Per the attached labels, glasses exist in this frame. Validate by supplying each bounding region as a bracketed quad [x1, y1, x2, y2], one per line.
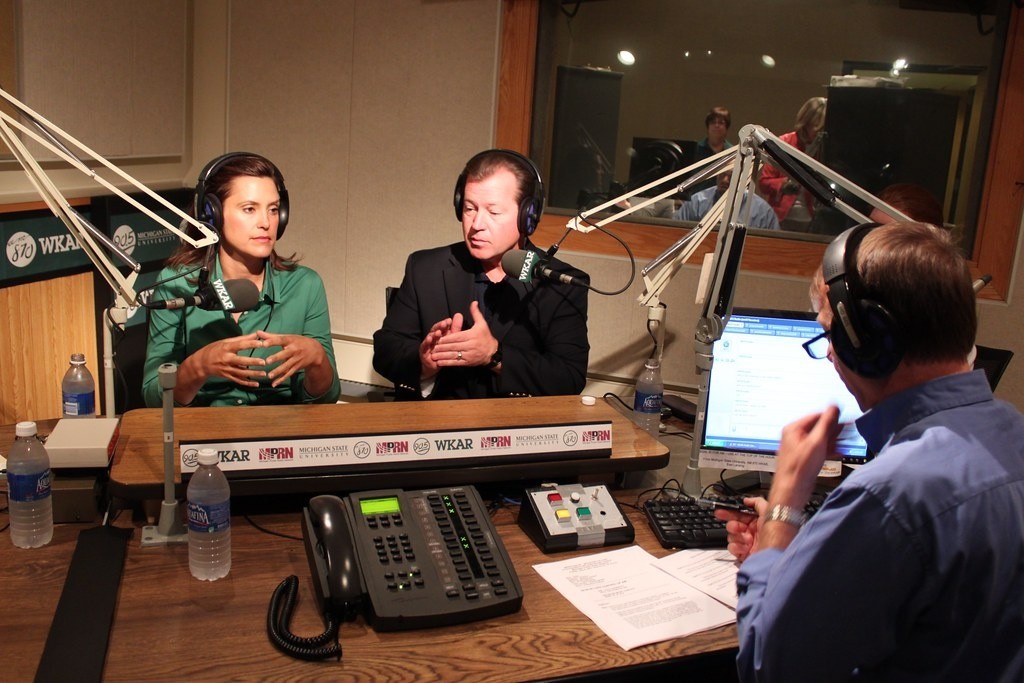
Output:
[802, 322, 834, 363]
[710, 120, 727, 125]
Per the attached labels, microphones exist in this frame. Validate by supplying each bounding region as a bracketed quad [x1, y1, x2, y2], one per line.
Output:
[149, 279, 261, 313]
[501, 250, 585, 287]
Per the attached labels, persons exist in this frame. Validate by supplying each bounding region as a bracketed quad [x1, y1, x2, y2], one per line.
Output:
[692, 105, 733, 193]
[672, 149, 781, 231]
[615, 194, 676, 220]
[372, 150, 591, 395]
[141, 150, 341, 409]
[758, 95, 828, 233]
[715, 222, 1024, 683]
[871, 181, 946, 229]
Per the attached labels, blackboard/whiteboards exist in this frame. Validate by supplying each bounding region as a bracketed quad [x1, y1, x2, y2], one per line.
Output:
[0, 0, 194, 206]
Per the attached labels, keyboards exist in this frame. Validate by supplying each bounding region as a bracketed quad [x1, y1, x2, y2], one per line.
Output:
[643, 494, 825, 550]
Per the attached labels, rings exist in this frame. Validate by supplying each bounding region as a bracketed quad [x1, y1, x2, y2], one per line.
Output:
[458, 351, 462, 360]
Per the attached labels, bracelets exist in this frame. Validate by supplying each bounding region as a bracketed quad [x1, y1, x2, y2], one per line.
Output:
[767, 503, 809, 528]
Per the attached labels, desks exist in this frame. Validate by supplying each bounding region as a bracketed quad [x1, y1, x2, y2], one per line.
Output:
[0, 396, 851, 683]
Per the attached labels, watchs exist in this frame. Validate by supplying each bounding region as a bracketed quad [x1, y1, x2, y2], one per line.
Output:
[487, 349, 501, 369]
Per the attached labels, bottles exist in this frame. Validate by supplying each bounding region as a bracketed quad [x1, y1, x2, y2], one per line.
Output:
[186, 448, 231, 581]
[631, 359, 663, 440]
[62, 354, 96, 419]
[7, 421, 53, 549]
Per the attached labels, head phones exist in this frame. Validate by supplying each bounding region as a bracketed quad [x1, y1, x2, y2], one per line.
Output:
[454, 149, 544, 237]
[821, 222, 908, 379]
[194, 151, 290, 241]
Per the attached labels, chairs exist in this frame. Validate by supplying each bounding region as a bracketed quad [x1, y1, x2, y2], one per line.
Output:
[114, 273, 153, 409]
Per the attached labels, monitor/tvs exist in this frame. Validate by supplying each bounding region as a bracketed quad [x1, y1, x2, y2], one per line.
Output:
[697, 306, 874, 498]
[629, 137, 698, 201]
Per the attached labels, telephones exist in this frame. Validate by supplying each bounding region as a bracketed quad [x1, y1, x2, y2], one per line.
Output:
[301, 484, 524, 632]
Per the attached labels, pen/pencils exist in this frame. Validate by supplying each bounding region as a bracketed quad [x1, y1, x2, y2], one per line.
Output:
[688, 497, 759, 516]
